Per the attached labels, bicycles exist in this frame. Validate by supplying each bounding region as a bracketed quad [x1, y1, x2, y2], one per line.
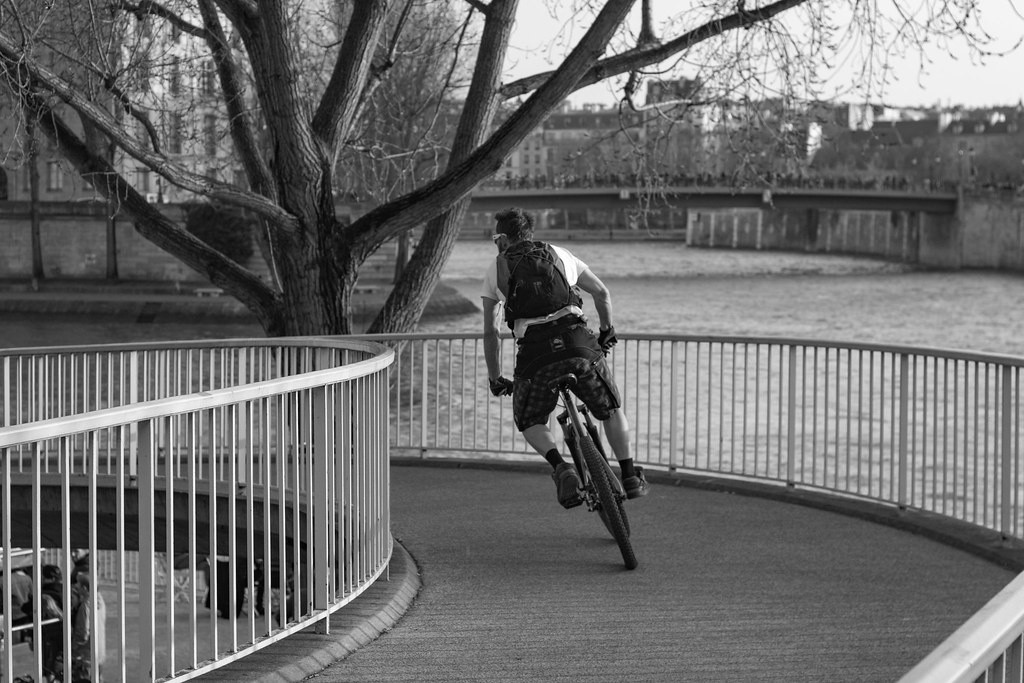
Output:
[500, 338, 639, 569]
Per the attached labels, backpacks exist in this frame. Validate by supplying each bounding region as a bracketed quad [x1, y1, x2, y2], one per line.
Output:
[503, 240, 584, 338]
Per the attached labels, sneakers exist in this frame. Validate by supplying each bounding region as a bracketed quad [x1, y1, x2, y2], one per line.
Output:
[622, 466, 648, 499]
[551, 462, 584, 510]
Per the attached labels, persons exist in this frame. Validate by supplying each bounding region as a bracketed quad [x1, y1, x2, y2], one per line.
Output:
[480, 206, 650, 508]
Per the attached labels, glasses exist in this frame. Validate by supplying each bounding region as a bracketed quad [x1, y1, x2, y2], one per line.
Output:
[492, 233, 508, 244]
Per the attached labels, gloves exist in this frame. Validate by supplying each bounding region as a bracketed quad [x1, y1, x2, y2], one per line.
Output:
[597, 326, 618, 354]
[489, 376, 513, 396]
